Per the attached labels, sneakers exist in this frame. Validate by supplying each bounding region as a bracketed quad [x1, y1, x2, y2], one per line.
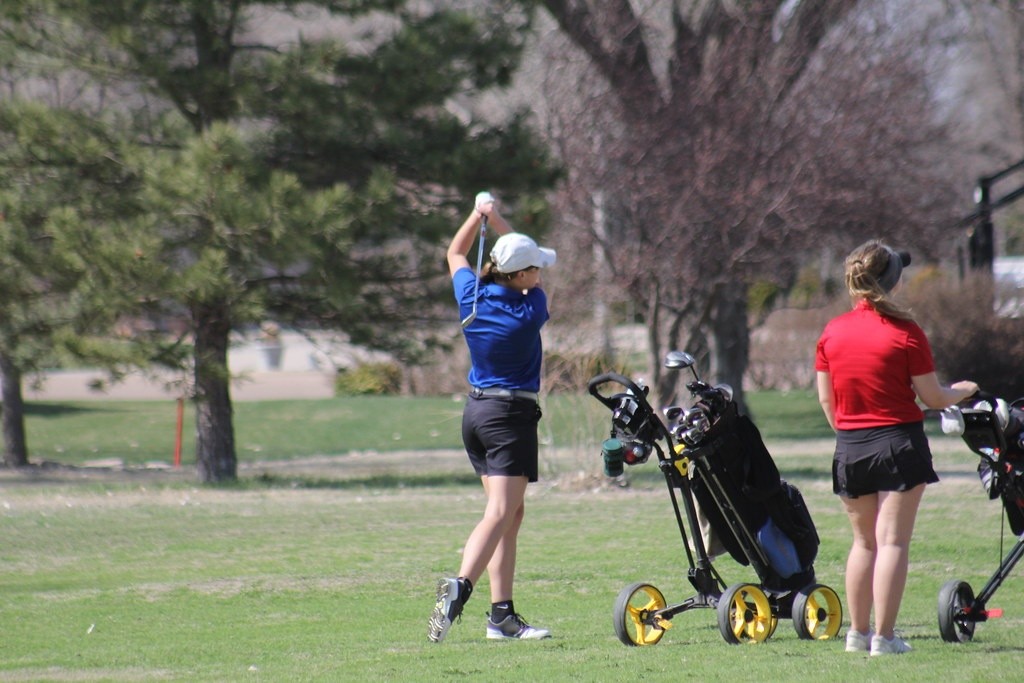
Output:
[845, 630, 876, 652]
[427, 577, 465, 644]
[485, 612, 552, 641]
[869, 635, 915, 656]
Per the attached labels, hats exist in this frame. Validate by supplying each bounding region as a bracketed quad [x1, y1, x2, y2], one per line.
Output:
[489, 233, 557, 273]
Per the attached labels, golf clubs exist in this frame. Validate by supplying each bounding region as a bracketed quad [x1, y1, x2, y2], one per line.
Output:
[460, 214, 488, 328]
[660, 351, 726, 446]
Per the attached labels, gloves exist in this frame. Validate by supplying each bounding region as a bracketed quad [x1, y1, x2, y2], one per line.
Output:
[474, 191, 495, 210]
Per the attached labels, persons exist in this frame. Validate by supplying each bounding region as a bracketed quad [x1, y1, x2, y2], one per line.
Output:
[425, 190, 558, 644]
[813, 239, 982, 658]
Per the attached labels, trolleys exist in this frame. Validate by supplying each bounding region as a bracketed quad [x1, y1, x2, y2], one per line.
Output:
[926, 384, 1024, 643]
[585, 374, 843, 649]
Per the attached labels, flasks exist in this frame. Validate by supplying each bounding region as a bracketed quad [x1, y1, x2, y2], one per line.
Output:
[602, 438, 624, 479]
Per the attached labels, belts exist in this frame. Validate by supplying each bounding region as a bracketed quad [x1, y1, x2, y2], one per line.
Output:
[470, 385, 539, 402]
